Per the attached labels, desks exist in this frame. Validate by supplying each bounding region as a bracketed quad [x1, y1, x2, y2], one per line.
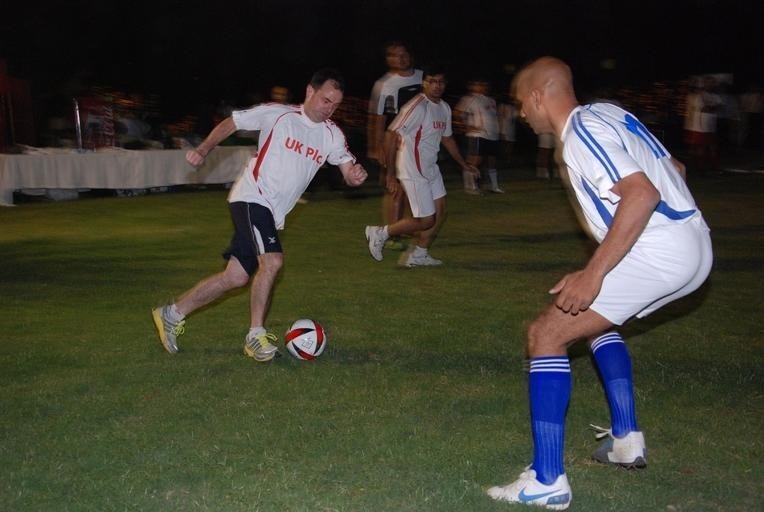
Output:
[0, 142, 255, 206]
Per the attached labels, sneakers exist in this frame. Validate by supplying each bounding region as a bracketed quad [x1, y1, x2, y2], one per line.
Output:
[151, 304, 185, 355]
[589, 424, 647, 471]
[365, 225, 385, 261]
[486, 463, 572, 510]
[406, 253, 443, 267]
[242, 334, 282, 362]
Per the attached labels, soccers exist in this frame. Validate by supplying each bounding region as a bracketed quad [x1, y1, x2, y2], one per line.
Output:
[283, 318, 327, 361]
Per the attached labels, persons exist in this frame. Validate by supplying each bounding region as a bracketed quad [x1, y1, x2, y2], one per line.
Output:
[364, 67, 482, 268]
[149, 67, 369, 363]
[364, 41, 426, 251]
[484, 57, 714, 510]
[452, 77, 504, 198]
[683, 76, 764, 177]
[268, 81, 289, 105]
[535, 133, 560, 179]
